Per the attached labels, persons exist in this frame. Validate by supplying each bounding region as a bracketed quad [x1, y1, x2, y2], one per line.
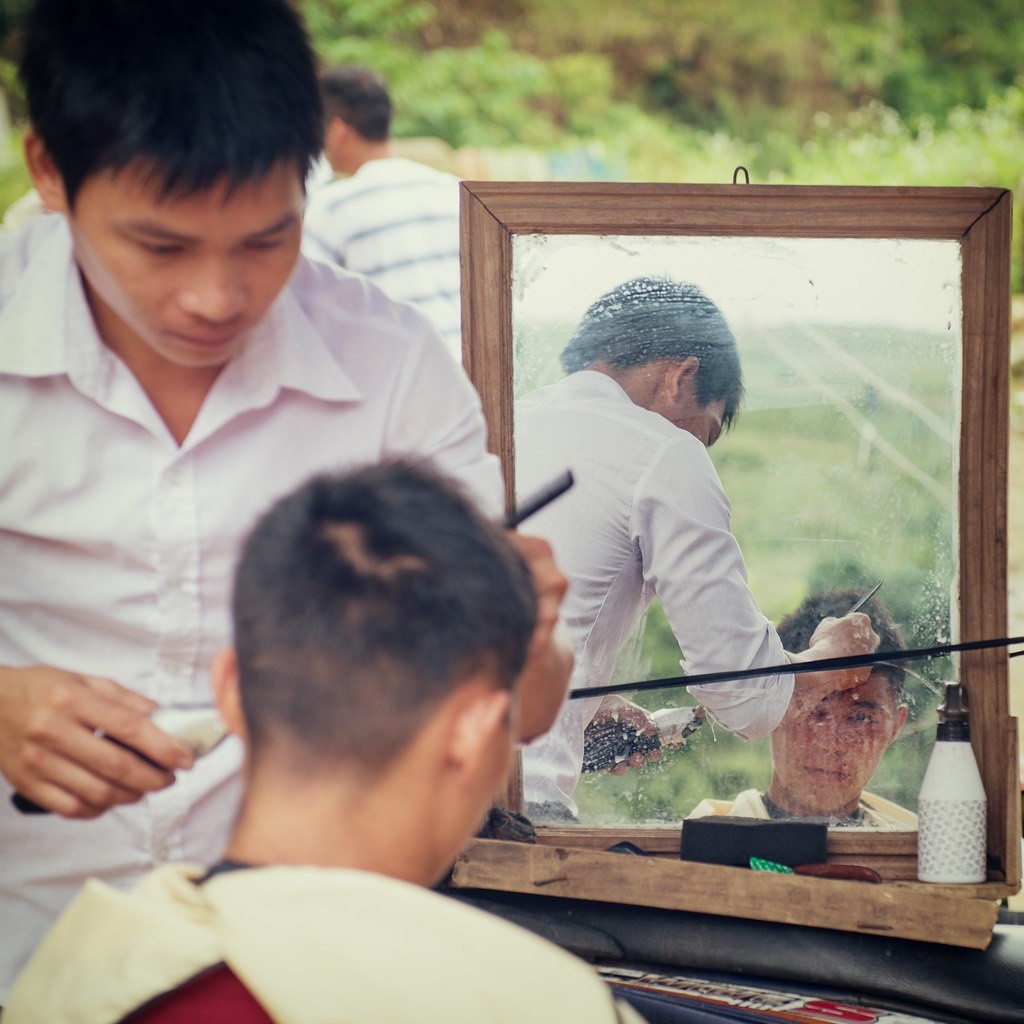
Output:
[684, 588, 912, 831]
[0, 0, 578, 1012]
[298, 64, 459, 364]
[1, 446, 636, 1024]
[514, 271, 883, 820]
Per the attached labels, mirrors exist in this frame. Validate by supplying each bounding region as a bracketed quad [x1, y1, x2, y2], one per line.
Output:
[457, 181, 1013, 881]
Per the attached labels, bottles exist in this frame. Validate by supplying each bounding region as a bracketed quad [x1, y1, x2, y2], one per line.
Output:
[917, 686, 989, 885]
